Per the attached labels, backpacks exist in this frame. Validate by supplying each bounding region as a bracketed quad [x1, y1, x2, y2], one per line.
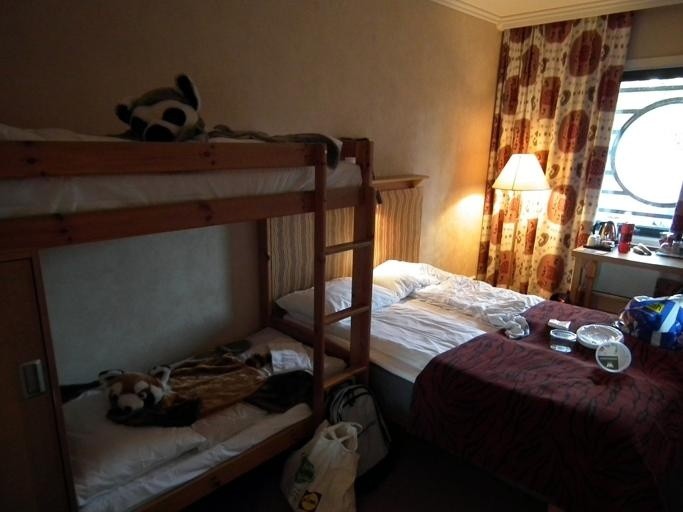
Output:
[324, 380, 392, 477]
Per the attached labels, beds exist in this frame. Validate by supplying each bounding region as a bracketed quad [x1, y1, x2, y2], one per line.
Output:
[0, 135, 378, 512]
[258, 175, 682, 511]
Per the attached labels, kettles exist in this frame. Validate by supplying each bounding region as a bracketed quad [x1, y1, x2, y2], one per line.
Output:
[592, 220, 619, 246]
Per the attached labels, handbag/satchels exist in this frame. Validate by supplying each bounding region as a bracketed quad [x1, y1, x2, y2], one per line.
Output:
[276, 420, 364, 512]
[611, 294, 683, 349]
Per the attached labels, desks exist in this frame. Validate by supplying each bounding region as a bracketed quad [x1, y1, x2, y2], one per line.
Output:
[568, 239, 682, 316]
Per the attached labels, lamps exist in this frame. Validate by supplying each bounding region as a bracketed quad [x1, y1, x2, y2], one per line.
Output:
[491, 153, 552, 290]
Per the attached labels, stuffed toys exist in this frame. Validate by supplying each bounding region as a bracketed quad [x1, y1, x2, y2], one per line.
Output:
[107, 72, 206, 143]
[97, 364, 204, 427]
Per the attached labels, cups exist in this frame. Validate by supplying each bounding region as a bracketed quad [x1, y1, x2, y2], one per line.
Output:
[586, 234, 615, 246]
[616, 222, 635, 253]
[548, 329, 577, 353]
[658, 243, 669, 253]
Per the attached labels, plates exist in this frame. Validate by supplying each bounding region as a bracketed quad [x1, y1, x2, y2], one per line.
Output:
[575, 324, 625, 350]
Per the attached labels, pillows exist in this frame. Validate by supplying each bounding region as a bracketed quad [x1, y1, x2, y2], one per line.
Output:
[274, 274, 400, 327]
[61, 387, 206, 506]
[373, 259, 452, 301]
